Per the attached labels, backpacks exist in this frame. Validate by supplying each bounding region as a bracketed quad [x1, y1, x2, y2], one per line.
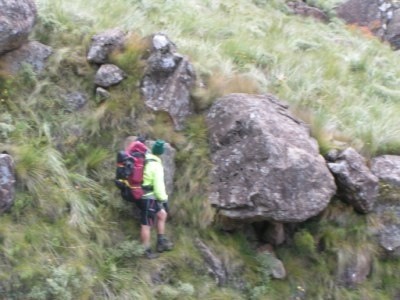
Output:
[117, 141, 147, 200]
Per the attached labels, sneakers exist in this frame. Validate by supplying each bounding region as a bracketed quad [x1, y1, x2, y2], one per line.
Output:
[144, 251, 159, 259]
[157, 241, 174, 252]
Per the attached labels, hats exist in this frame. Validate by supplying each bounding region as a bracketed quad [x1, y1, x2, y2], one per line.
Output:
[152, 140, 165, 154]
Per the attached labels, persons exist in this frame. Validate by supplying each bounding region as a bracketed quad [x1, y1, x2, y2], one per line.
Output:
[142, 141, 175, 260]
